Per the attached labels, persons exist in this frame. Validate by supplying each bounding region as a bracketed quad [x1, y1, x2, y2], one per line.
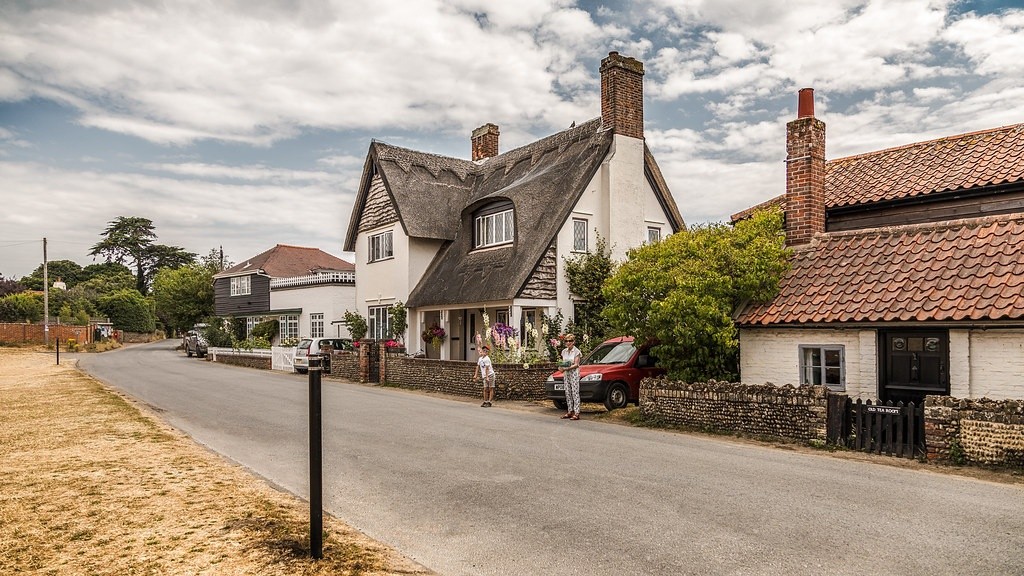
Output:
[558, 333, 583, 420]
[472, 345, 495, 407]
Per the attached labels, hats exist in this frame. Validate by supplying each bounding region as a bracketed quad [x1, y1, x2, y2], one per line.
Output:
[481, 344, 491, 350]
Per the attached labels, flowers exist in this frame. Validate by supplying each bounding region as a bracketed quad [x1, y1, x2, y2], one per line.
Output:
[385, 338, 404, 347]
[489, 323, 516, 348]
[421, 323, 447, 353]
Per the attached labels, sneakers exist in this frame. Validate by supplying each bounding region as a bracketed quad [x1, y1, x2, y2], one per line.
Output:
[481, 401, 492, 407]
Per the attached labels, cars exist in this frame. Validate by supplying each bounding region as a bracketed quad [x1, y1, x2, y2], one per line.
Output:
[99, 327, 110, 338]
[543, 334, 668, 412]
[293, 337, 352, 374]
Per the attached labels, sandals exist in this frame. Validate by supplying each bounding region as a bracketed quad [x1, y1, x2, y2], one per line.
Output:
[570, 413, 580, 420]
[560, 412, 572, 419]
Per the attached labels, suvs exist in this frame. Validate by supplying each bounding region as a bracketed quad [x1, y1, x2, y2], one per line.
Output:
[185, 330, 211, 358]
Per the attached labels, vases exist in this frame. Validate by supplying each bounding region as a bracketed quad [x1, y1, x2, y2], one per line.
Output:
[387, 347, 405, 352]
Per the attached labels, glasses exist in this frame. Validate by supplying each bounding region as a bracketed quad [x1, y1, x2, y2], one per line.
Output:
[565, 340, 574, 342]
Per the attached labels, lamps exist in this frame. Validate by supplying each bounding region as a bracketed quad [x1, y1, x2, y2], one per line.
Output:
[458, 315, 462, 326]
